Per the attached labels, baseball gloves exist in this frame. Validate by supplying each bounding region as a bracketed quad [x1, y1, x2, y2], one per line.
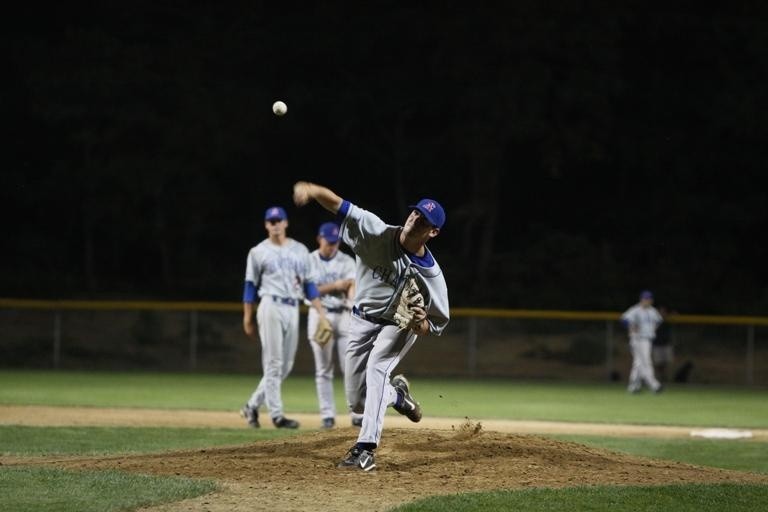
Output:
[313, 318, 334, 347]
[392, 279, 426, 332]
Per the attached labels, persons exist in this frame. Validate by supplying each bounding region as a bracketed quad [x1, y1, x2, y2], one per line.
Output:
[304, 217, 363, 432]
[617, 288, 668, 396]
[290, 177, 451, 473]
[651, 304, 681, 384]
[238, 203, 334, 430]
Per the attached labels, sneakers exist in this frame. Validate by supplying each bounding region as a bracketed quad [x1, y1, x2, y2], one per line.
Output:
[337, 443, 378, 472]
[350, 416, 362, 427]
[239, 404, 262, 429]
[321, 417, 335, 428]
[390, 373, 422, 423]
[271, 416, 300, 430]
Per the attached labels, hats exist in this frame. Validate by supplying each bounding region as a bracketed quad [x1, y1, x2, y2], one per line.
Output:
[318, 222, 341, 243]
[408, 199, 446, 229]
[640, 291, 653, 299]
[264, 206, 287, 221]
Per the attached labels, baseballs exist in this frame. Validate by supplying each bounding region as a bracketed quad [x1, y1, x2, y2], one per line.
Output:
[273, 101, 288, 117]
